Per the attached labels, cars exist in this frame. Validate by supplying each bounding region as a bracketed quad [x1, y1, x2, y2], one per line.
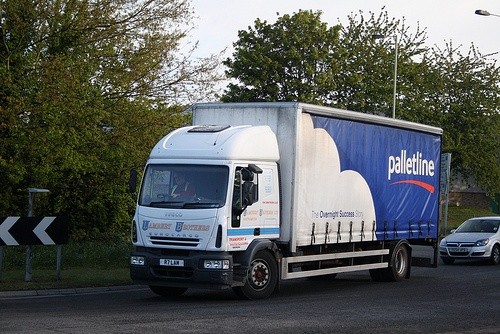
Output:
[440, 216, 500, 265]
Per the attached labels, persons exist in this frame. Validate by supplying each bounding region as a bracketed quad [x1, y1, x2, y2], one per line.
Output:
[168, 171, 195, 199]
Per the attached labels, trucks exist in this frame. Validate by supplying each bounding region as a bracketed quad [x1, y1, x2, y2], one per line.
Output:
[132, 103, 442, 302]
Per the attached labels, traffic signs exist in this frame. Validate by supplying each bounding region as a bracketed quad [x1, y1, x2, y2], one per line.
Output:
[0, 216, 70, 245]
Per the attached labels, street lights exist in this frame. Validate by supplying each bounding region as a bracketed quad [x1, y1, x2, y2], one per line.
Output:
[372, 33, 400, 119]
